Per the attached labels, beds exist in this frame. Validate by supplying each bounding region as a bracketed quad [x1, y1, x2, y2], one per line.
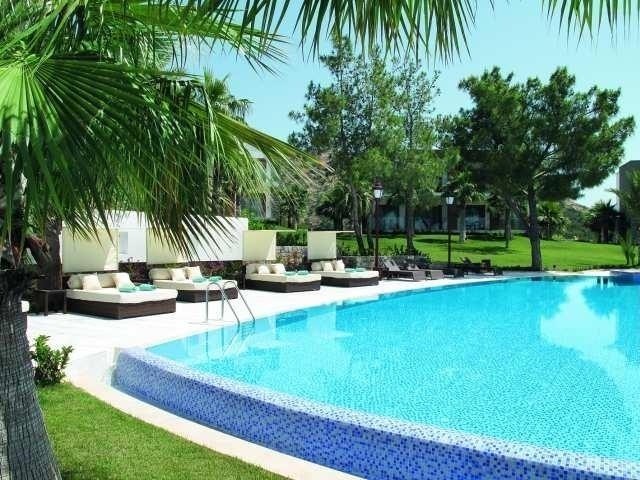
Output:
[148, 267, 238, 303]
[62, 270, 179, 319]
[309, 261, 379, 287]
[244, 263, 321, 293]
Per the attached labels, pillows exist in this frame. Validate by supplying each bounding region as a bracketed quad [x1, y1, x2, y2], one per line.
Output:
[320, 260, 344, 272]
[255, 263, 286, 274]
[167, 266, 202, 282]
[77, 271, 137, 292]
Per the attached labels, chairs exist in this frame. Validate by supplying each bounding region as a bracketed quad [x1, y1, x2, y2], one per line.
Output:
[382, 255, 445, 282]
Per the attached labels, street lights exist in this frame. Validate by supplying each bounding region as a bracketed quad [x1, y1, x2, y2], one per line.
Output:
[370, 178, 385, 282]
[442, 190, 457, 274]
